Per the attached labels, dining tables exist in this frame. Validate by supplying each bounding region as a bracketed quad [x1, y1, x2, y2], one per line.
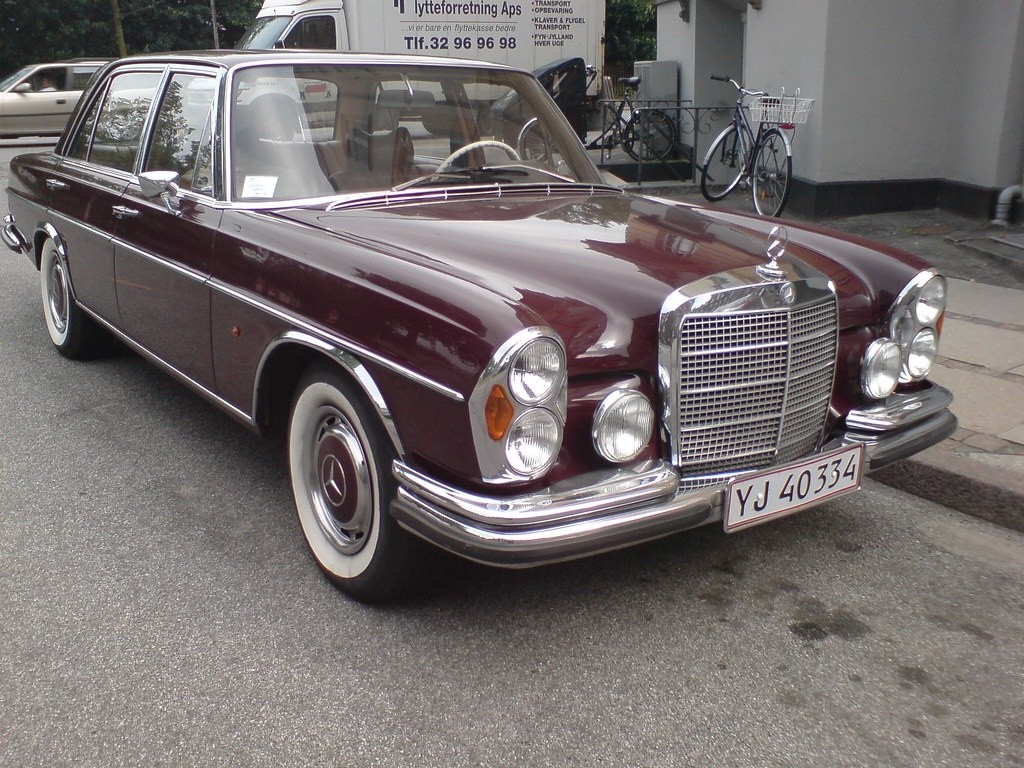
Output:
[349, 127, 422, 191]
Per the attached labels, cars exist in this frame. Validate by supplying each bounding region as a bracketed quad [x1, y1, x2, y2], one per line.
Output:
[2, 46, 962, 603]
[0, 59, 157, 141]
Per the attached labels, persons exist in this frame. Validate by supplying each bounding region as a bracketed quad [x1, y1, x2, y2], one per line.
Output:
[38, 73, 58, 92]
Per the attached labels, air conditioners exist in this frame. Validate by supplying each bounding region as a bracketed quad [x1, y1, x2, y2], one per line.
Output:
[633, 60, 678, 158]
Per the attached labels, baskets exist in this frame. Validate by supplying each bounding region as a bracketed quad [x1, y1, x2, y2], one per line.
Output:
[747, 94, 813, 124]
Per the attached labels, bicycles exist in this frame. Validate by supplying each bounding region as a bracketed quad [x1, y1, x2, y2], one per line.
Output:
[518, 62, 677, 168]
[700, 73, 813, 217]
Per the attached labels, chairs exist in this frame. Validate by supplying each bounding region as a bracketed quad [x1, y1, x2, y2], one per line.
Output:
[237, 106, 299, 197]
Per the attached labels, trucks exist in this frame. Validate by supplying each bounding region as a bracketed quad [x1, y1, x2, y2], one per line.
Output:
[189, 0, 609, 161]
